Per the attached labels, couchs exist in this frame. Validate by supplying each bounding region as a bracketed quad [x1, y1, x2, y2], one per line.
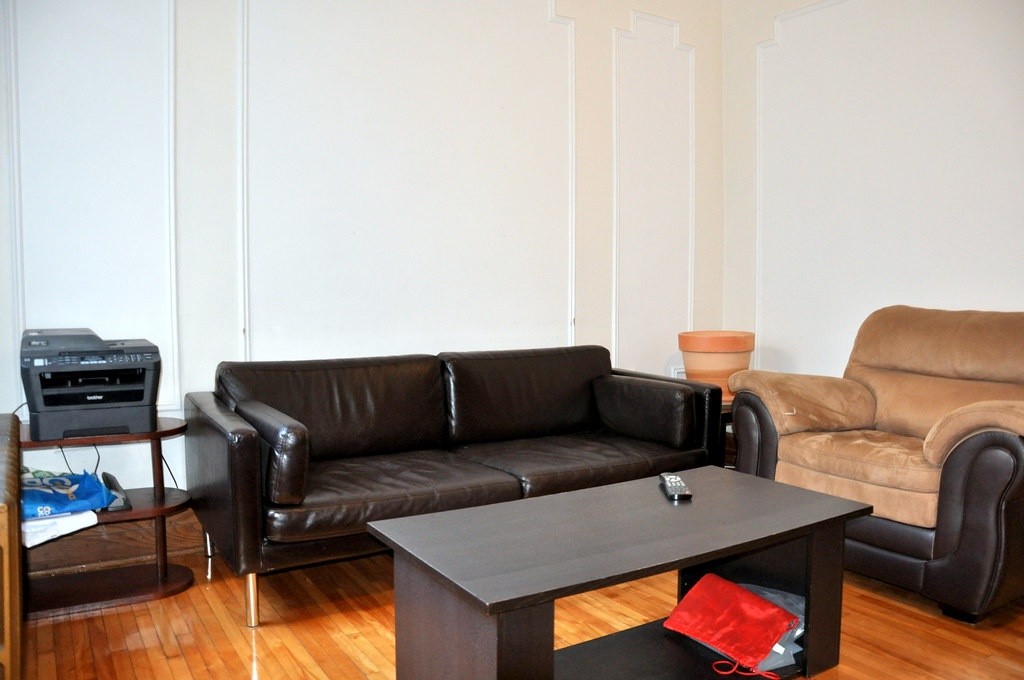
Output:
[728, 305, 1024, 626]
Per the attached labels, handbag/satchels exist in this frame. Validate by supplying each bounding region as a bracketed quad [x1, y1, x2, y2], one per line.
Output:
[663, 572, 806, 680]
[19, 466, 118, 548]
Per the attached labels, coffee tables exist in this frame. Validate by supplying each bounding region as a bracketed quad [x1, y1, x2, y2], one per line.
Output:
[361, 465, 875, 680]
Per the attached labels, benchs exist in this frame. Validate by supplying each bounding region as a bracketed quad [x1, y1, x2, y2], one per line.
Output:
[183, 344, 726, 628]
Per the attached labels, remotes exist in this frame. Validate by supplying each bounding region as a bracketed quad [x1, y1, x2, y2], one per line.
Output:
[659, 472, 692, 501]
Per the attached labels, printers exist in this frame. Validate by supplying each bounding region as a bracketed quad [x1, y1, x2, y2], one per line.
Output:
[21, 328, 162, 442]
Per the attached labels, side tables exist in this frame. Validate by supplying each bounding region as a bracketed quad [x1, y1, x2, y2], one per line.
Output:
[18, 416, 195, 622]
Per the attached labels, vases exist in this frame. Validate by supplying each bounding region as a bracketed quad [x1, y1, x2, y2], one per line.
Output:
[678, 331, 756, 404]
